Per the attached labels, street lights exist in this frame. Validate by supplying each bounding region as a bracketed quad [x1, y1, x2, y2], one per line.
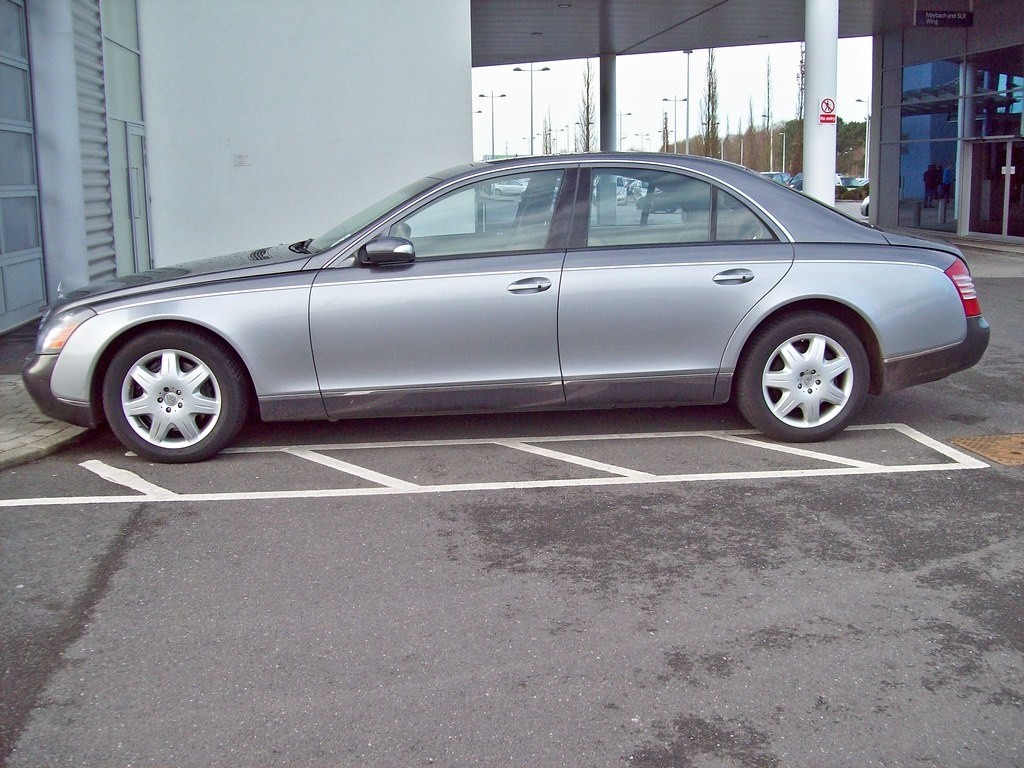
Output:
[684, 49, 694, 153]
[635, 133, 648, 150]
[703, 122, 721, 156]
[574, 122, 580, 153]
[549, 128, 564, 153]
[662, 99, 688, 153]
[566, 125, 570, 152]
[763, 115, 773, 172]
[616, 113, 632, 151]
[479, 94, 506, 158]
[514, 66, 551, 155]
[546, 133, 551, 154]
[646, 138, 649, 149]
[779, 133, 786, 172]
[658, 130, 673, 153]
[523, 137, 536, 156]
[855, 98, 870, 178]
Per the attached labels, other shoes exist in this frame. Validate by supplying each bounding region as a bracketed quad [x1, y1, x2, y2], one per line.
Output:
[923, 205, 927, 208]
[929, 205, 934, 208]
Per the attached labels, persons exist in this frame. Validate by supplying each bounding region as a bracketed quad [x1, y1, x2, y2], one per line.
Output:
[924, 164, 943, 208]
[943, 161, 953, 204]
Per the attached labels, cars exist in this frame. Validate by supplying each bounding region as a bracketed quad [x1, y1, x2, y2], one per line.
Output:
[624, 178, 662, 197]
[636, 188, 685, 213]
[524, 178, 561, 199]
[835, 173, 869, 191]
[23, 147, 992, 462]
[861, 196, 870, 216]
[491, 179, 529, 194]
[593, 176, 628, 204]
[757, 170, 802, 191]
